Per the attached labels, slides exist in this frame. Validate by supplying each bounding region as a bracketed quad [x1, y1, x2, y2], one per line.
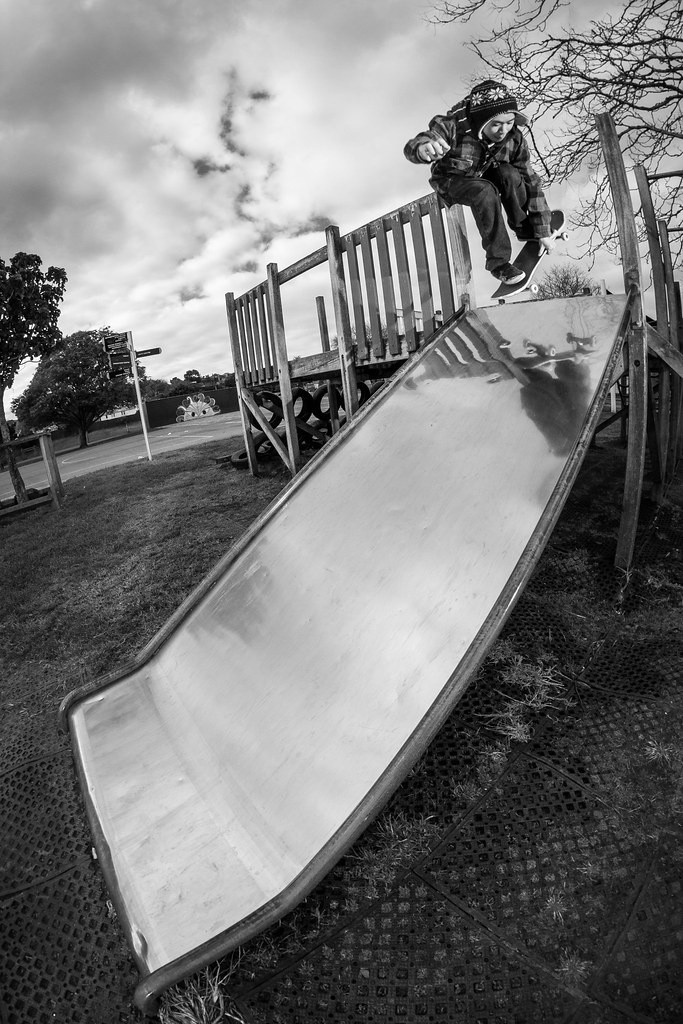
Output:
[60, 295, 634, 1012]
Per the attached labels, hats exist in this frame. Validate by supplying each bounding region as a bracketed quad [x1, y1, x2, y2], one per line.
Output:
[469, 80, 550, 189]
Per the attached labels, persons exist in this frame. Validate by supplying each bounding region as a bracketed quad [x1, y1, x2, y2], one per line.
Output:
[405, 79, 551, 285]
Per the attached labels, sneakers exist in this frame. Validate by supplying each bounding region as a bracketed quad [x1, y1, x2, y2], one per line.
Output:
[491, 262, 526, 285]
[517, 227, 557, 241]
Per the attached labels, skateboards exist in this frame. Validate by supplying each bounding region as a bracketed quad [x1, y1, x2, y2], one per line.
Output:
[492, 208, 568, 300]
